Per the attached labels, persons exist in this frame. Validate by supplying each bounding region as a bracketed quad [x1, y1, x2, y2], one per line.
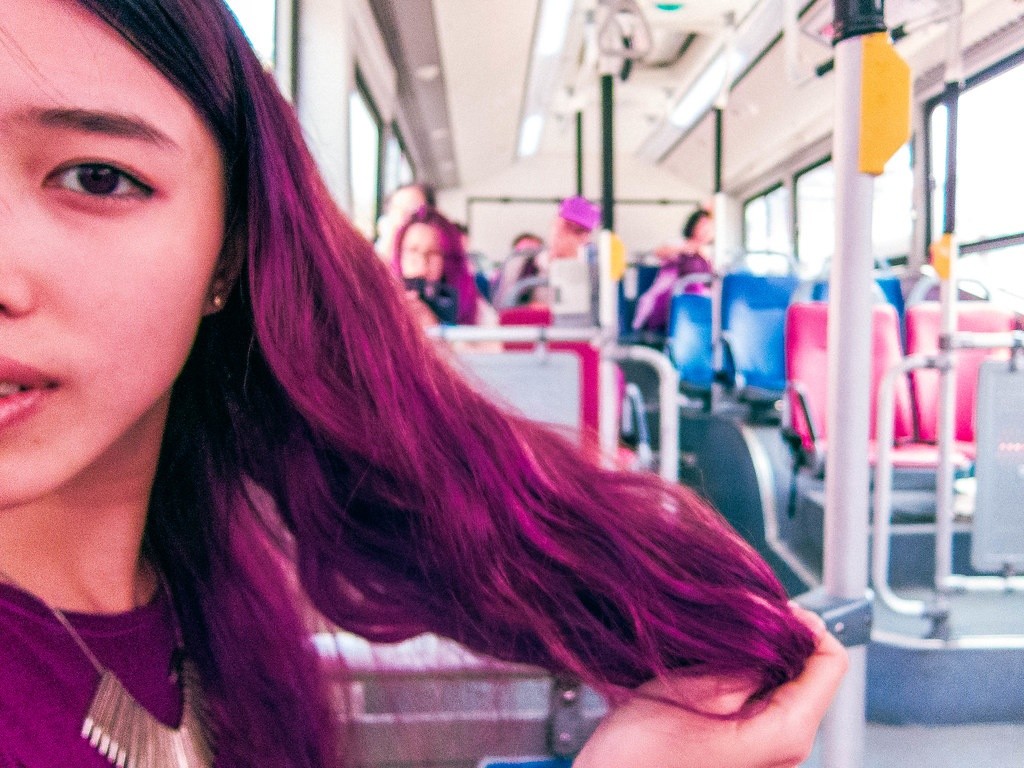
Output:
[0, 0, 847, 768]
[371, 184, 436, 264]
[682, 210, 713, 261]
[396, 208, 498, 327]
[493, 196, 614, 306]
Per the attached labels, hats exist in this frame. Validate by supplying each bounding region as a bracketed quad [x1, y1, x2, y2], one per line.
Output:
[559, 196, 601, 230]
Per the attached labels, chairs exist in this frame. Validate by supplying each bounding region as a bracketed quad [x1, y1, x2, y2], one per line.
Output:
[312, 248, 1023, 768]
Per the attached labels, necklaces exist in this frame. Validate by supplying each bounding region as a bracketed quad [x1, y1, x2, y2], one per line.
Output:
[0, 570, 219, 768]
